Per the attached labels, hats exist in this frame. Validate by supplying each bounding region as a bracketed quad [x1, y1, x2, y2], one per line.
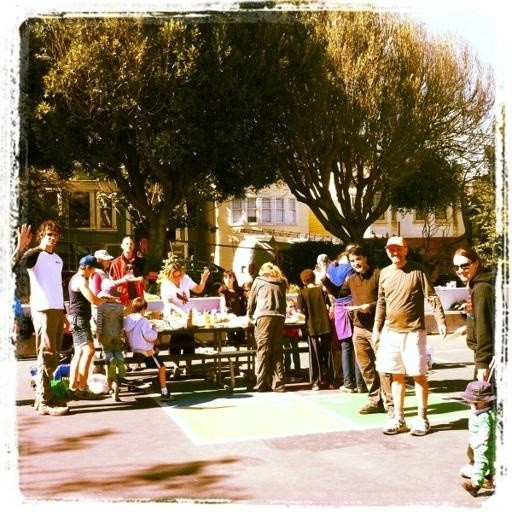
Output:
[79, 255, 103, 269]
[459, 380, 496, 403]
[385, 235, 407, 248]
[94, 249, 115, 261]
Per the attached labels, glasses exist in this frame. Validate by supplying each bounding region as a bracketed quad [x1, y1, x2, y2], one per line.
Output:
[450, 260, 475, 270]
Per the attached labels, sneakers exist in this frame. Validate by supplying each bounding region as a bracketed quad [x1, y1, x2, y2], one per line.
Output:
[339, 385, 355, 393]
[312, 385, 320, 391]
[482, 479, 493, 488]
[70, 386, 104, 401]
[383, 420, 409, 435]
[329, 384, 336, 389]
[410, 418, 431, 436]
[460, 463, 474, 479]
[459, 477, 480, 497]
[388, 405, 394, 418]
[34, 398, 70, 416]
[173, 367, 180, 379]
[160, 393, 176, 401]
[357, 403, 386, 414]
[184, 368, 195, 378]
[356, 387, 368, 393]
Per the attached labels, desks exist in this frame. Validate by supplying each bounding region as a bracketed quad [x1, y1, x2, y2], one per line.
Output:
[70, 310, 312, 371]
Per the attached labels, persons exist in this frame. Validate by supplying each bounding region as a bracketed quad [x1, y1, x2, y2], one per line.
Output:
[371, 237, 448, 436]
[458, 375, 495, 497]
[452, 247, 495, 479]
[11, 220, 69, 415]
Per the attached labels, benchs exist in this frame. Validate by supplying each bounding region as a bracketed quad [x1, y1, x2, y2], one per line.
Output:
[92, 344, 309, 402]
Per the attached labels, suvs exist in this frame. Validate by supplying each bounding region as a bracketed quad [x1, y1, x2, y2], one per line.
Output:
[129, 256, 238, 288]
